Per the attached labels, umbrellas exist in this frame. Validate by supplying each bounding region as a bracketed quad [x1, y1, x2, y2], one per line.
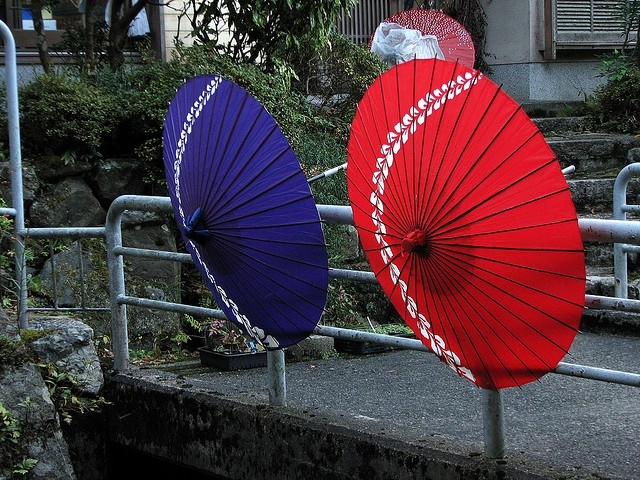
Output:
[347, 54, 590, 393]
[367, 9, 476, 73]
[161, 73, 330, 350]
